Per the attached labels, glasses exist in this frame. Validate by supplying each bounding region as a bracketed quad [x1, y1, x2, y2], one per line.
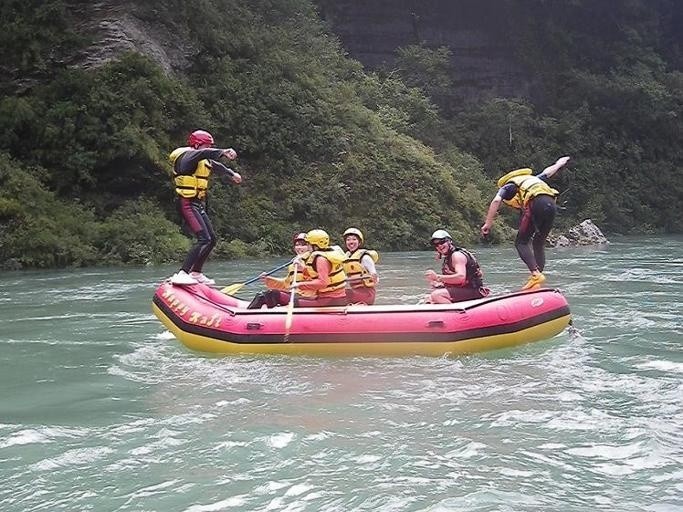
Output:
[433, 239, 449, 247]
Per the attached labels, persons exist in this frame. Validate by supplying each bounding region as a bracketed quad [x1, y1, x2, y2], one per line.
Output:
[481, 156, 570, 290]
[170, 130, 241, 284]
[248, 229, 349, 309]
[424, 229, 483, 305]
[259, 234, 316, 298]
[342, 228, 379, 305]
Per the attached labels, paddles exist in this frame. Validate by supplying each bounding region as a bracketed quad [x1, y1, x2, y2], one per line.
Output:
[284, 263, 297, 329]
[218, 262, 292, 296]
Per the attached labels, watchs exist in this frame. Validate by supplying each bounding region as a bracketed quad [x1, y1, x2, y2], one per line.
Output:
[436, 275, 441, 283]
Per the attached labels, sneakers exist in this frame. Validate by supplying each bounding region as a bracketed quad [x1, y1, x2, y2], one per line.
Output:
[189, 272, 216, 285]
[169, 272, 198, 285]
[522, 272, 546, 291]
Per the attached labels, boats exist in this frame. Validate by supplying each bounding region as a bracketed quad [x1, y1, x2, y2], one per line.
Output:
[151, 274, 572, 360]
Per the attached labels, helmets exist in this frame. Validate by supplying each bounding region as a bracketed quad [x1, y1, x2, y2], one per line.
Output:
[430, 230, 453, 240]
[303, 229, 330, 250]
[187, 129, 214, 147]
[343, 227, 364, 245]
[293, 232, 307, 242]
